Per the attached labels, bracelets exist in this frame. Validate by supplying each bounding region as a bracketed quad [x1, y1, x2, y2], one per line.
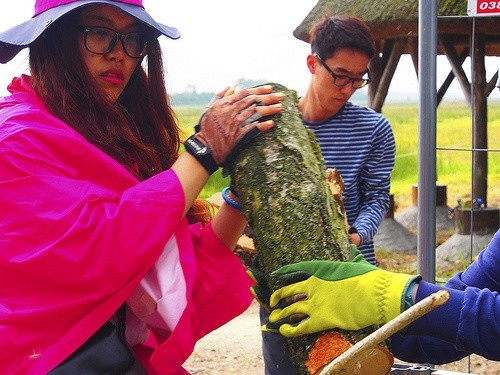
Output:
[230, 187, 242, 200]
[222, 186, 244, 213]
[183, 135, 219, 176]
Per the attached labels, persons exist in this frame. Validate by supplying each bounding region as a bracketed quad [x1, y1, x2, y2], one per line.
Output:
[261, 226, 500, 364]
[260, 15, 395, 375]
[0, 0, 285, 375]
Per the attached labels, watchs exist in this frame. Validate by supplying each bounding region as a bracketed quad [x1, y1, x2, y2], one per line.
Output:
[404, 281, 416, 309]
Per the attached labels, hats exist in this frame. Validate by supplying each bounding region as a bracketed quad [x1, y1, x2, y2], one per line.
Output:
[0, 0, 181, 64]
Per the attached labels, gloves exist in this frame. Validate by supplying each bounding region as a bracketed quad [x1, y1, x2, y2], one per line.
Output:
[246, 267, 279, 334]
[268, 243, 423, 338]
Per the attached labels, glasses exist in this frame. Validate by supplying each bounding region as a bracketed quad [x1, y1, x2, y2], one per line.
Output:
[76, 24, 157, 59]
[313, 54, 372, 89]
